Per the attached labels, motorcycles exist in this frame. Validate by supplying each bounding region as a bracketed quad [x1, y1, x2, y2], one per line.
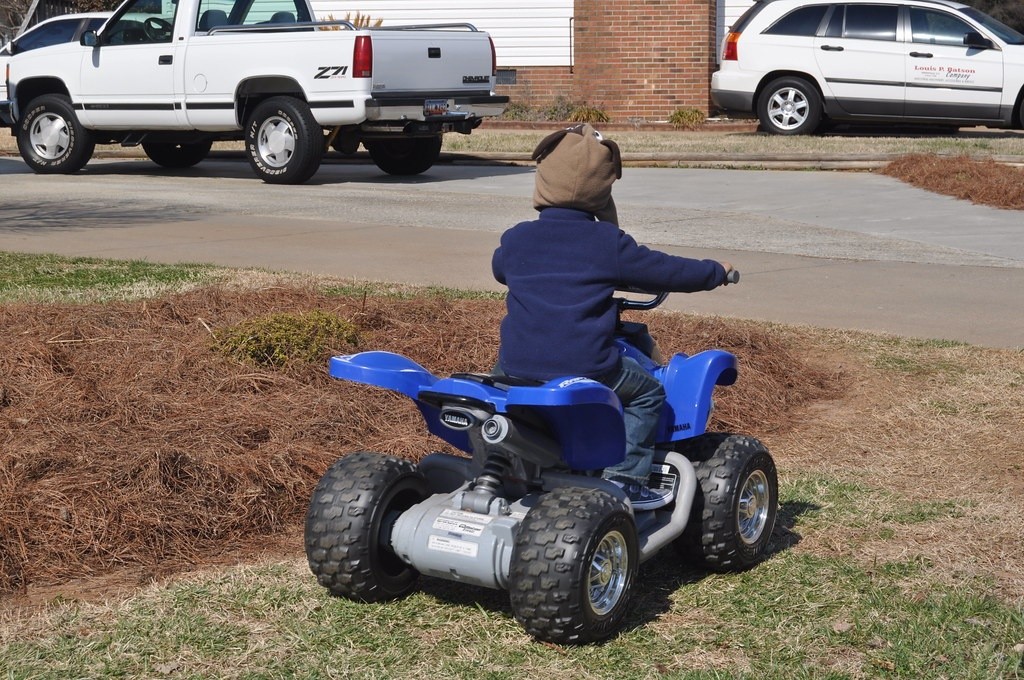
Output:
[300, 267, 780, 646]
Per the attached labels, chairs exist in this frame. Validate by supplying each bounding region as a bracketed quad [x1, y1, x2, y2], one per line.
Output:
[270, 11, 296, 23]
[199, 10, 228, 31]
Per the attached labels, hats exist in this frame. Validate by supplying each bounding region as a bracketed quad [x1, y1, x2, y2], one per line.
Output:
[531, 123, 622, 229]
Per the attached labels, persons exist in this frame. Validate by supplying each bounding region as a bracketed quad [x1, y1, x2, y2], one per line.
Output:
[492, 123, 735, 512]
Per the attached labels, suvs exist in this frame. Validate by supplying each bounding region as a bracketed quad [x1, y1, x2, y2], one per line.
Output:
[709, 0, 1024, 137]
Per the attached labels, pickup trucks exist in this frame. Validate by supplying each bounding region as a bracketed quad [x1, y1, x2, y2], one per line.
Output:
[0, 0, 513, 187]
